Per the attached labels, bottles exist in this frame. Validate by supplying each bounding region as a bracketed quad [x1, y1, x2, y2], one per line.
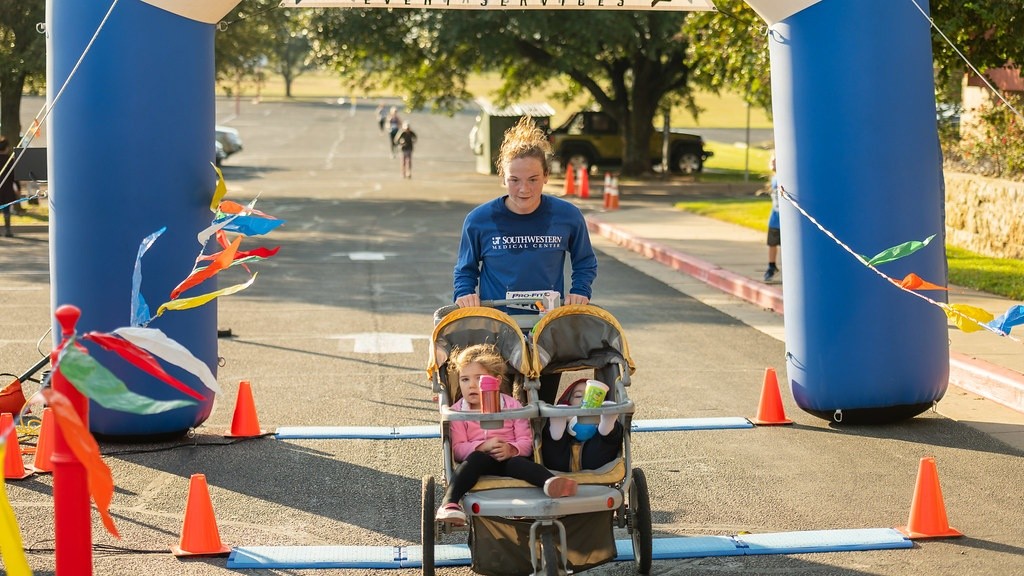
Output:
[480, 374, 501, 413]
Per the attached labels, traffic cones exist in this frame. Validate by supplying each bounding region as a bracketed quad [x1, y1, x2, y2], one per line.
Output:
[563, 163, 576, 197]
[169, 474, 232, 556]
[747, 367, 793, 425]
[577, 168, 593, 199]
[894, 456, 964, 538]
[601, 173, 619, 209]
[25, 406, 55, 473]
[224, 381, 266, 438]
[0, 413, 36, 479]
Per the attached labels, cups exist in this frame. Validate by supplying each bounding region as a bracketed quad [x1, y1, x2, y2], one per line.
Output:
[581, 380, 609, 409]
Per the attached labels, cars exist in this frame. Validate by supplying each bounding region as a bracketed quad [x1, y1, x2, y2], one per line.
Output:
[548, 111, 714, 176]
[216, 125, 241, 166]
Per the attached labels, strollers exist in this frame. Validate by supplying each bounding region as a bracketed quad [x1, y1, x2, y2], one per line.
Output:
[422, 297, 653, 576]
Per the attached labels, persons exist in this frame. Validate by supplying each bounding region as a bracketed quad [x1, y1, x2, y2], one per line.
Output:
[0, 137, 25, 238]
[436, 344, 577, 526]
[395, 122, 417, 178]
[541, 381, 622, 472]
[386, 108, 401, 159]
[376, 103, 386, 132]
[761, 153, 781, 282]
[453, 125, 597, 308]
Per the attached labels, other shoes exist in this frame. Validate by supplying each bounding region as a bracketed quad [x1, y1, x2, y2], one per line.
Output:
[763, 268, 775, 281]
[543, 477, 577, 497]
[436, 503, 467, 526]
